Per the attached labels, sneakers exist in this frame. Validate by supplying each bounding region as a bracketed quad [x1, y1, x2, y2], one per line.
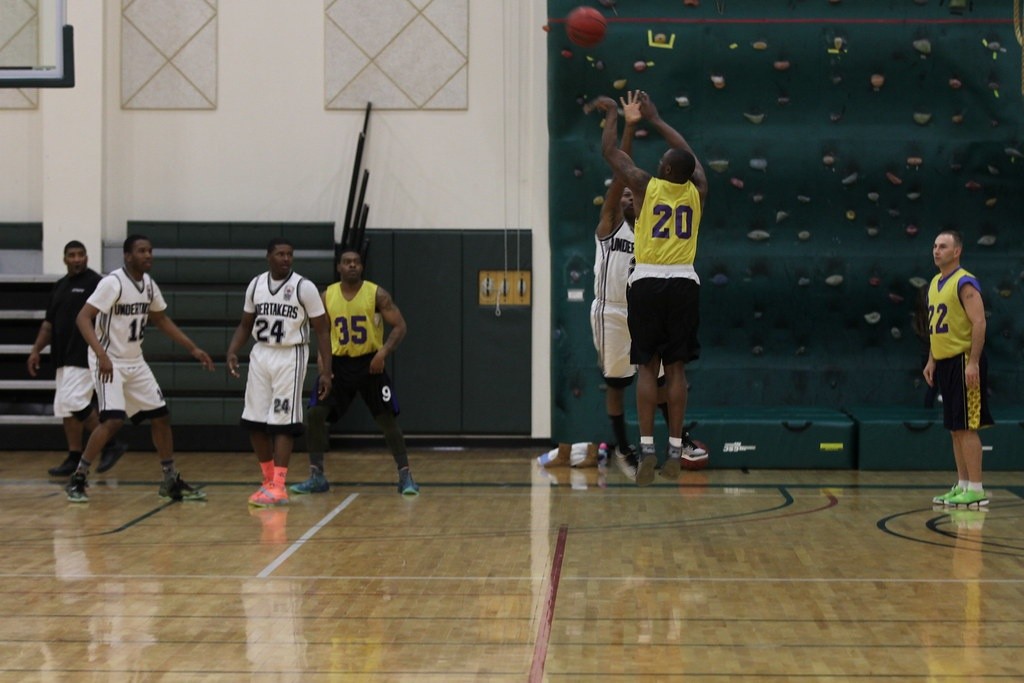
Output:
[680, 431, 709, 461]
[635, 443, 658, 487]
[289, 465, 329, 493]
[614, 445, 638, 484]
[247, 477, 288, 507]
[397, 471, 419, 494]
[932, 480, 989, 507]
[64, 471, 90, 503]
[658, 445, 682, 481]
[158, 471, 207, 499]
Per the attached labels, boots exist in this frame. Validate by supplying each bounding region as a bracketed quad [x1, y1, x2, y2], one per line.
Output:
[544, 442, 572, 467]
[577, 444, 598, 468]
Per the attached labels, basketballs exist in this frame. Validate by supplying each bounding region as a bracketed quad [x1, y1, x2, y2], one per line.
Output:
[566, 6, 607, 48]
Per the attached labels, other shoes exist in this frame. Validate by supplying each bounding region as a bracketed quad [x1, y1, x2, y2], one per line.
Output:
[48, 458, 79, 475]
[97, 438, 128, 473]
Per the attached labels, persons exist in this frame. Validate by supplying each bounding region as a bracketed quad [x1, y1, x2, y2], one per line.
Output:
[226, 236, 333, 509]
[65, 233, 215, 503]
[922, 229, 994, 508]
[286, 246, 421, 494]
[28, 241, 128, 477]
[591, 90, 711, 479]
